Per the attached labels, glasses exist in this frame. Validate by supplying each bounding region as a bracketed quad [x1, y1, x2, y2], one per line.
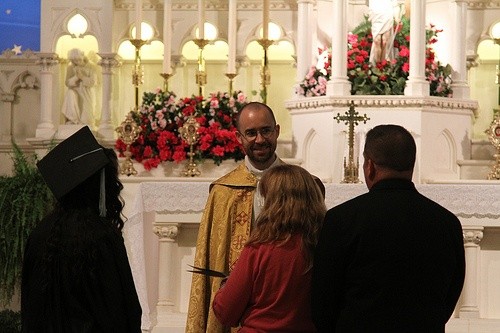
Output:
[238, 123, 276, 142]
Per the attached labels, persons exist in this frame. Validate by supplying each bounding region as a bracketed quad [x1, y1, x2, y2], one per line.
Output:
[184, 101, 328, 333]
[365, 0, 407, 74]
[62, 48, 104, 134]
[211, 164, 329, 333]
[20, 124, 144, 333]
[302, 122, 466, 333]
